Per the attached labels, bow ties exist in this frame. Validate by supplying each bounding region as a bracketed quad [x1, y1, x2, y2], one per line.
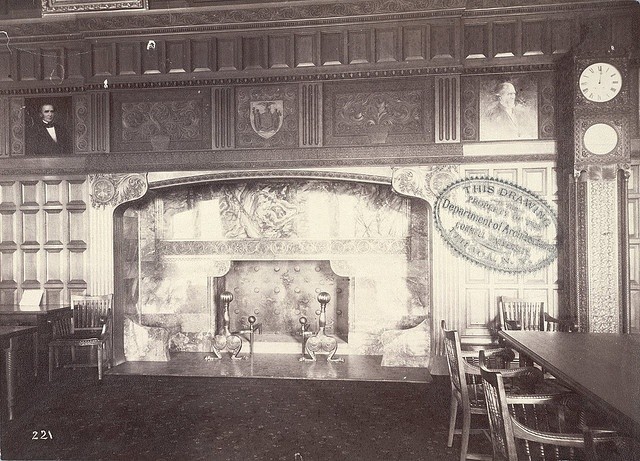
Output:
[42, 122, 55, 128]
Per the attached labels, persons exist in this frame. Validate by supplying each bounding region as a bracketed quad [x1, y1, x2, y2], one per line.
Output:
[30, 103, 68, 154]
[483, 82, 534, 140]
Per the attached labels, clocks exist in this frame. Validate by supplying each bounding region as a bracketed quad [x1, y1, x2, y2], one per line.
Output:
[579, 61, 624, 102]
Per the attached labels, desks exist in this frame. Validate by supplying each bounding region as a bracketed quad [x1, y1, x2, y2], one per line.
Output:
[499, 330, 639, 459]
[1, 323, 40, 421]
[3, 302, 71, 374]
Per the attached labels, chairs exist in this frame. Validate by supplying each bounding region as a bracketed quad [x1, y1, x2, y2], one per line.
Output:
[499, 296, 576, 367]
[584, 427, 640, 461]
[49, 294, 113, 381]
[440, 321, 546, 460]
[479, 349, 618, 459]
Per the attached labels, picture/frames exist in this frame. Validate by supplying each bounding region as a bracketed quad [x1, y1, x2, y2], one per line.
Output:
[478, 75, 543, 142]
[24, 97, 75, 157]
